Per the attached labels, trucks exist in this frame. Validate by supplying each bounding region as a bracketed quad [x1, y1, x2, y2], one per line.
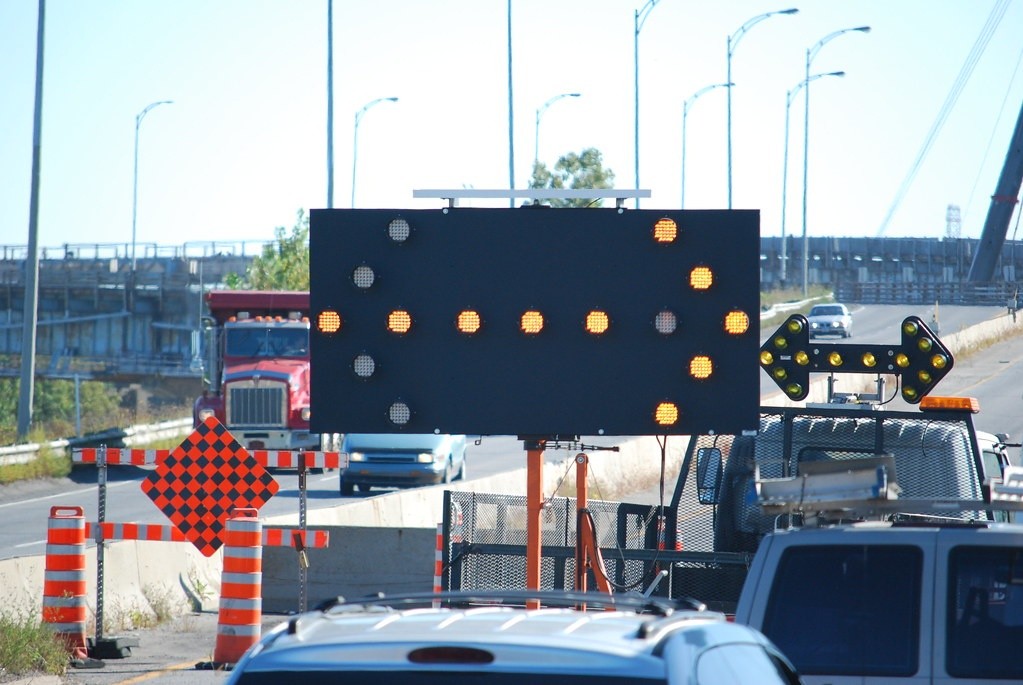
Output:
[662, 378, 1023, 615]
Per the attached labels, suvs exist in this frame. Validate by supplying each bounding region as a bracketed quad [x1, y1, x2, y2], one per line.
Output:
[225, 589, 811, 684]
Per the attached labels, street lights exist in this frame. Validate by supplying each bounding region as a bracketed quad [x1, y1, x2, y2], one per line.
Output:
[131, 100, 177, 270]
[803, 25, 873, 300]
[728, 7, 799, 210]
[780, 71, 846, 280]
[351, 96, 399, 208]
[681, 83, 737, 214]
[532, 92, 583, 187]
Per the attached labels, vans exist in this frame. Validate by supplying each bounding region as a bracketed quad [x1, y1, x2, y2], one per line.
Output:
[732, 454, 1023, 684]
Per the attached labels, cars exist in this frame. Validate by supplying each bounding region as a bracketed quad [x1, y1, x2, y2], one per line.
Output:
[338, 433, 469, 497]
[807, 302, 852, 339]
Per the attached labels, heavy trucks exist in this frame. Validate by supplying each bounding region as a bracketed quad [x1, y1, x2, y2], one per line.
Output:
[189, 260, 346, 477]
